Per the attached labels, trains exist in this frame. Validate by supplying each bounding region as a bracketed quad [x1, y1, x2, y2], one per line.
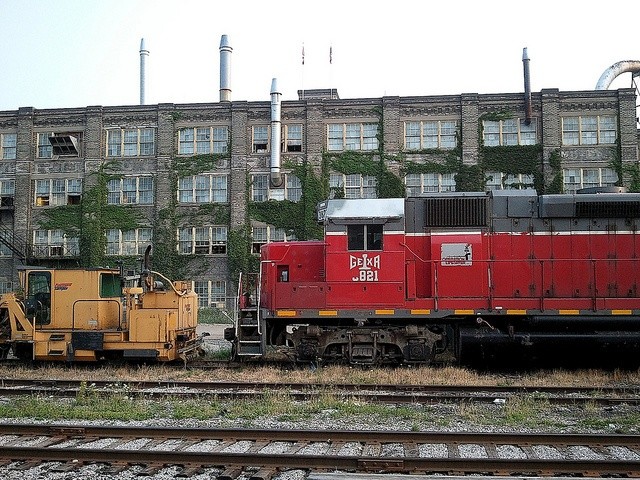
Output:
[0, 185, 640, 374]
